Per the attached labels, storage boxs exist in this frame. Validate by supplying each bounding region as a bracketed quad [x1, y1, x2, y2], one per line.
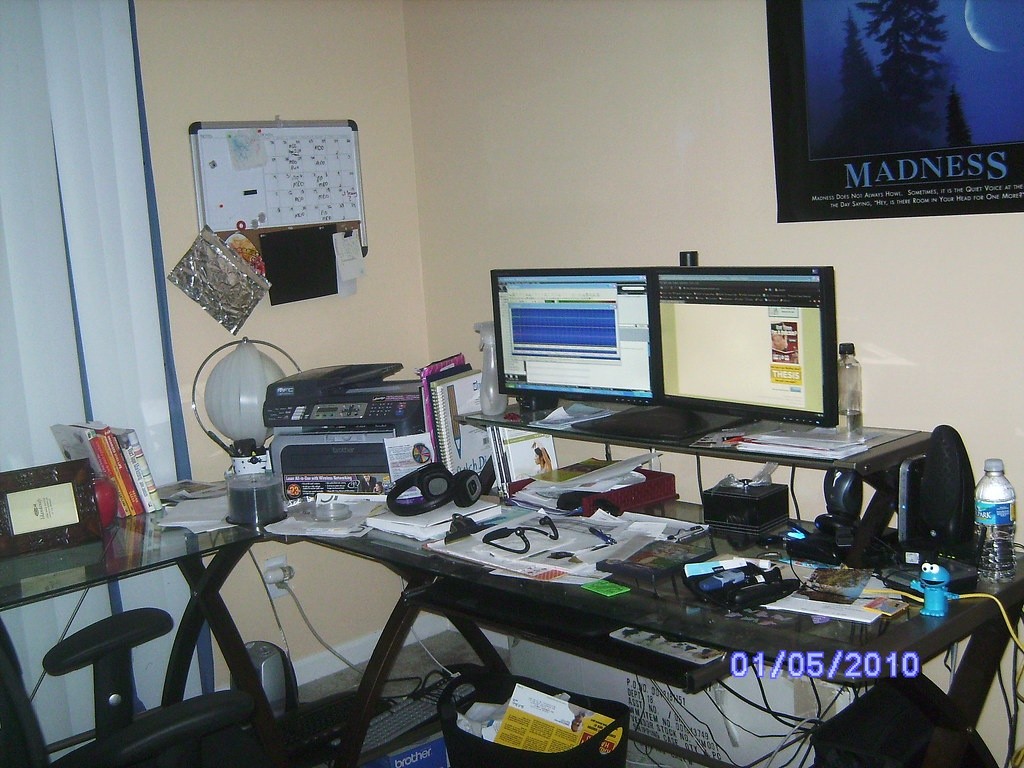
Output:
[509, 468, 677, 515]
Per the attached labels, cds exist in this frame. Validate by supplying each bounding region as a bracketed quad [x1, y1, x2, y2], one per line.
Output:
[226, 473, 284, 523]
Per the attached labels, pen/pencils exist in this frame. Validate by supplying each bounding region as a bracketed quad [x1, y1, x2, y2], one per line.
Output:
[587, 526, 616, 545]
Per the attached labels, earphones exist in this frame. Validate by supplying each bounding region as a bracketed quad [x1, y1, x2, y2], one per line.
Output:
[515, 526, 525, 536]
[539, 516, 551, 525]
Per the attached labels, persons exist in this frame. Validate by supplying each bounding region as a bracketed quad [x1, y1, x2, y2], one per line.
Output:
[359, 475, 383, 493]
[531, 442, 552, 474]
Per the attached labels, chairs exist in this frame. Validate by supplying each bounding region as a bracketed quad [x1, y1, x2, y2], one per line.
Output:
[0, 606, 269, 768]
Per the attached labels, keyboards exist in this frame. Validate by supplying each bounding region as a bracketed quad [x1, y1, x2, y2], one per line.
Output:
[354, 676, 478, 766]
[274, 684, 392, 768]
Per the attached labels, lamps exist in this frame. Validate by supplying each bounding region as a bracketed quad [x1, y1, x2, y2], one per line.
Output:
[193, 336, 301, 453]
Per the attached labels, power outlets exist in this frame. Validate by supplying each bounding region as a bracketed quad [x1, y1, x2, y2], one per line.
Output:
[266, 555, 292, 599]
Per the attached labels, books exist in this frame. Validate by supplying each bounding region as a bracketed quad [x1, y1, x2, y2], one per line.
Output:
[529, 457, 622, 484]
[51, 420, 162, 517]
[482, 423, 559, 499]
[419, 352, 492, 475]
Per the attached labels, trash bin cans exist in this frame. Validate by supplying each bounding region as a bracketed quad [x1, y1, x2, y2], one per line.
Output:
[437, 670, 631, 768]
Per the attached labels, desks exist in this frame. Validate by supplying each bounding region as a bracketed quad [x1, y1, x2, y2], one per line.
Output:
[0, 525, 290, 768]
[304, 408, 1024, 768]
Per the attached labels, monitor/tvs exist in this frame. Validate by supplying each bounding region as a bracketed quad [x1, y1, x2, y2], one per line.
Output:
[490, 267, 838, 442]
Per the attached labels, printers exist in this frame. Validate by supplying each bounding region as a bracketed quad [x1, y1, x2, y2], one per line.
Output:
[263, 362, 422, 504]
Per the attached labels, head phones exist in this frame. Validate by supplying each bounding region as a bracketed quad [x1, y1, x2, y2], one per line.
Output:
[386, 460, 482, 516]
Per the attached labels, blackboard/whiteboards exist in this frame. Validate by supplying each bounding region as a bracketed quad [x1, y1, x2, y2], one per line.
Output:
[188, 119, 370, 260]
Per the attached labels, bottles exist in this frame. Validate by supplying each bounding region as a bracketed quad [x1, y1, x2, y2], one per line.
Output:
[973, 458, 1017, 584]
[835, 342, 863, 439]
[480, 321, 508, 416]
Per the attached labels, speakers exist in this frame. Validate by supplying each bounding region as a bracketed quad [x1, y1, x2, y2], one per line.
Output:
[918, 424, 976, 545]
[231, 641, 300, 717]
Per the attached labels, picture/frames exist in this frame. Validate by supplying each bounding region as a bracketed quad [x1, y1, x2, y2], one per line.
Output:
[0, 457, 105, 559]
